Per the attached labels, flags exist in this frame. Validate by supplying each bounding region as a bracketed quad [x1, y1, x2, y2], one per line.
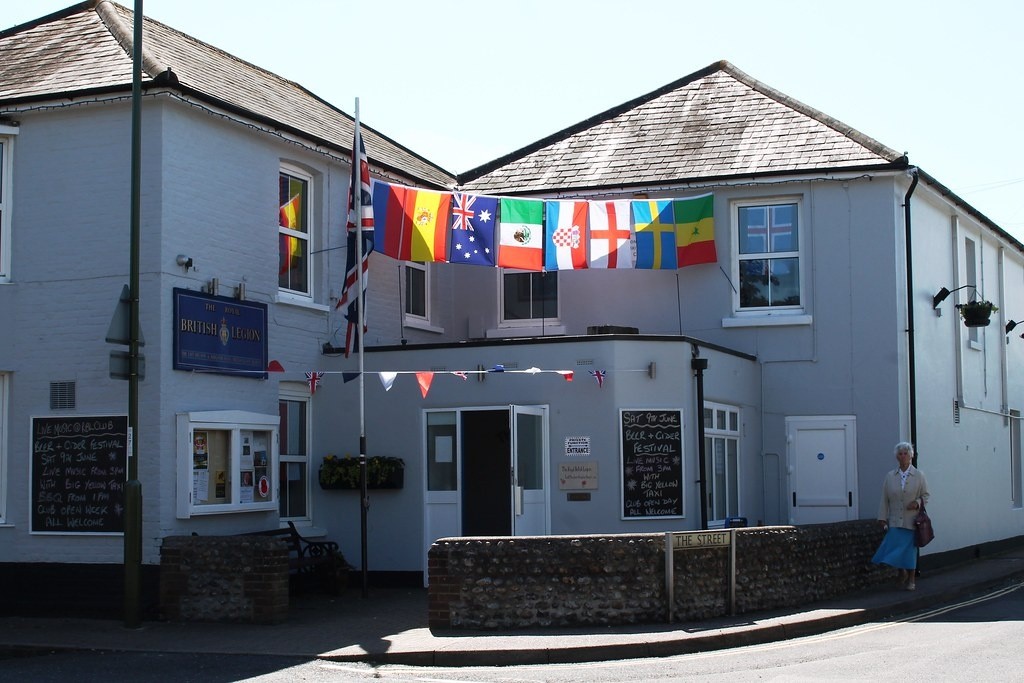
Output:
[369, 176, 719, 272]
[335, 96, 373, 360]
[746, 205, 793, 276]
[279, 193, 303, 276]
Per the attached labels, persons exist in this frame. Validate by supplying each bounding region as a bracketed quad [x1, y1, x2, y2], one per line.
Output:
[871, 441, 930, 591]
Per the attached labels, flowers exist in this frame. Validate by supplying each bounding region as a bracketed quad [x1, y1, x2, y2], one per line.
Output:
[319, 454, 406, 486]
[955, 301, 999, 319]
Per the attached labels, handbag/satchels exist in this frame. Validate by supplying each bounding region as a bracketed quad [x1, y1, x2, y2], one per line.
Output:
[913, 495, 935, 548]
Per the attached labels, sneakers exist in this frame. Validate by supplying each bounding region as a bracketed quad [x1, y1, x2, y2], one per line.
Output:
[905, 582, 917, 592]
[895, 573, 908, 587]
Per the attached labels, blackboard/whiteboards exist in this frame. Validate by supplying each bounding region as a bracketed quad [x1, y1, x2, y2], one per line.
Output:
[28, 412, 129, 536]
[618, 407, 686, 520]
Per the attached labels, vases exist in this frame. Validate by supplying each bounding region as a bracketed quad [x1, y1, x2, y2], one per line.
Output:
[317, 468, 403, 490]
[962, 307, 991, 327]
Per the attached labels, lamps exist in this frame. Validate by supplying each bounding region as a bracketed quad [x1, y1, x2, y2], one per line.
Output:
[478, 364, 486, 382]
[207, 277, 218, 296]
[234, 283, 246, 301]
[649, 361, 657, 378]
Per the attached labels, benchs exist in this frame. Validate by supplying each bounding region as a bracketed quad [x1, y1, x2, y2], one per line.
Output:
[191, 520, 340, 594]
